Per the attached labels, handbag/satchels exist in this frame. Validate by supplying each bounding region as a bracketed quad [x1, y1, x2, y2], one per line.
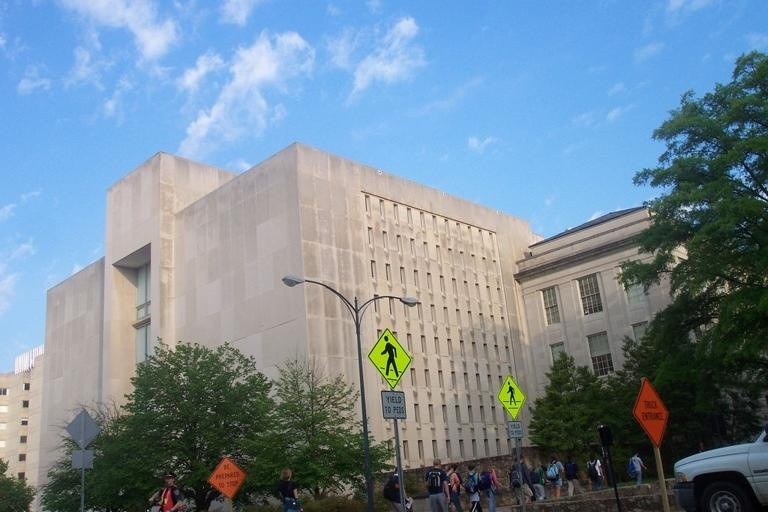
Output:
[283, 496, 298, 509]
[627, 465, 636, 478]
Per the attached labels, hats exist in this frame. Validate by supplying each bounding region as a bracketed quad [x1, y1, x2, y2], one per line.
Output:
[164, 471, 176, 479]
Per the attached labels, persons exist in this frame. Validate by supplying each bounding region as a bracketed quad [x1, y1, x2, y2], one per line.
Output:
[277, 468, 303, 512]
[148, 471, 184, 512]
[425, 453, 605, 512]
[629, 450, 648, 485]
[392, 466, 410, 512]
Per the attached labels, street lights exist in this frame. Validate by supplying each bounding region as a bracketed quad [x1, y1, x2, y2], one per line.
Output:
[280, 270, 420, 511]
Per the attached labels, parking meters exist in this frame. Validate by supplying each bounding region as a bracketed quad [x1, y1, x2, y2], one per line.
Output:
[594, 423, 623, 512]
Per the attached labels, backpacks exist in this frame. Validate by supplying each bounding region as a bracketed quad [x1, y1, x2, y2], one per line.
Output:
[510, 470, 521, 488]
[464, 472, 479, 494]
[383, 477, 398, 500]
[427, 468, 443, 491]
[546, 460, 559, 480]
[446, 472, 457, 492]
[589, 460, 598, 479]
[567, 463, 576, 480]
[478, 471, 493, 491]
[530, 472, 540, 483]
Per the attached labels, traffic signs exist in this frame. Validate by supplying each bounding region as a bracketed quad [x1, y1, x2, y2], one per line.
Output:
[377, 389, 409, 424]
[504, 422, 527, 440]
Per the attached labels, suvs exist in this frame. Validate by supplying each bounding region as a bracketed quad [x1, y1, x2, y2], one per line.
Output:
[670, 423, 766, 512]
